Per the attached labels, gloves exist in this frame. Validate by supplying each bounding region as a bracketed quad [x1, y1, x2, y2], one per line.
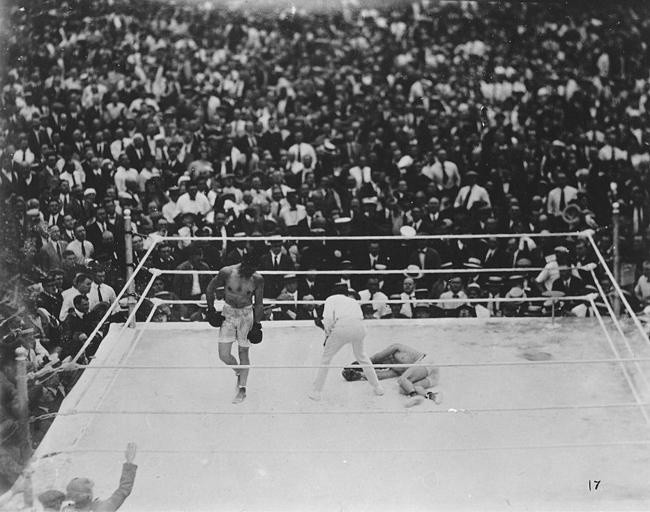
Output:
[342, 362, 363, 381]
[207, 306, 225, 327]
[246, 322, 263, 344]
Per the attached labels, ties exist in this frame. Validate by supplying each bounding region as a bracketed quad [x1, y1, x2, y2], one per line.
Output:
[189, 226, 194, 243]
[102, 222, 105, 231]
[369, 293, 375, 300]
[408, 296, 415, 315]
[373, 259, 376, 268]
[72, 173, 76, 186]
[311, 311, 315, 320]
[56, 243, 62, 263]
[23, 151, 26, 161]
[79, 199, 84, 209]
[492, 294, 498, 316]
[98, 145, 102, 153]
[121, 140, 125, 151]
[82, 241, 86, 256]
[137, 132, 650, 223]
[63, 194, 67, 207]
[52, 216, 56, 226]
[71, 231, 75, 242]
[274, 256, 279, 267]
[11, 170, 16, 185]
[97, 285, 104, 303]
[80, 142, 83, 152]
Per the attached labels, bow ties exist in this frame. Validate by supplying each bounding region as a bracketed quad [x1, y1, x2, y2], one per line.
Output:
[452, 292, 459, 297]
[418, 249, 427, 255]
[107, 215, 116, 220]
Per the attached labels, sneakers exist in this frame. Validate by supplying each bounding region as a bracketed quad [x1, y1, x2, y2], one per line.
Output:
[403, 395, 424, 407]
[236, 373, 241, 389]
[428, 391, 442, 405]
[373, 385, 385, 396]
[307, 391, 322, 401]
[232, 386, 247, 405]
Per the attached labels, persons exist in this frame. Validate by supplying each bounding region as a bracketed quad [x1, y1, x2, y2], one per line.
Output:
[0, 0, 650, 511]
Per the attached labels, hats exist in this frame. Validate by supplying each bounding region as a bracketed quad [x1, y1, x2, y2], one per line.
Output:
[516, 257, 534, 270]
[400, 224, 417, 241]
[504, 287, 528, 304]
[310, 224, 329, 236]
[264, 234, 289, 247]
[197, 292, 209, 309]
[485, 276, 506, 287]
[508, 272, 525, 283]
[402, 264, 425, 279]
[370, 262, 389, 277]
[230, 232, 253, 242]
[385, 294, 405, 308]
[462, 256, 484, 268]
[12, 326, 68, 383]
[281, 274, 298, 281]
[537, 229, 603, 316]
